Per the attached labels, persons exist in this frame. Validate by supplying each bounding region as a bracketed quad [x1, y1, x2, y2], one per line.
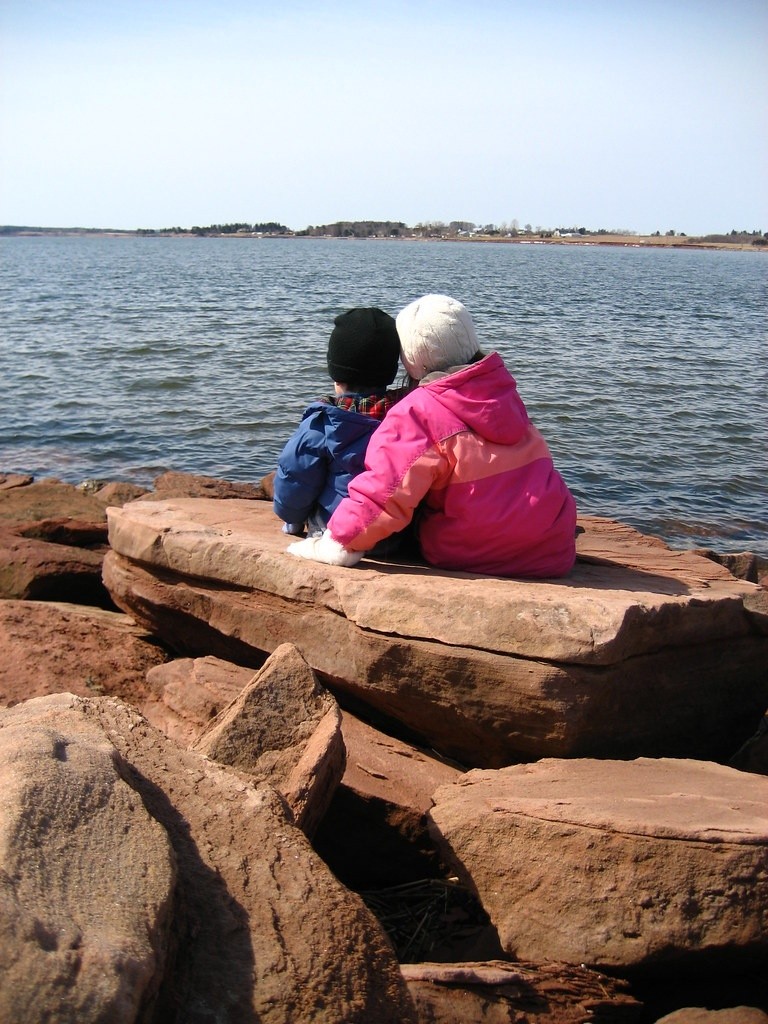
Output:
[286, 294, 577, 579]
[271, 306, 407, 555]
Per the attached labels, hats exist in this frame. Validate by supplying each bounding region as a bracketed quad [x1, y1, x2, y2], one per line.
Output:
[396, 293, 480, 381]
[327, 308, 400, 387]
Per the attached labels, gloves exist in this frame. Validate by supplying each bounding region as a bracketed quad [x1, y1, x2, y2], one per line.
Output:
[282, 523, 305, 533]
[288, 529, 365, 565]
